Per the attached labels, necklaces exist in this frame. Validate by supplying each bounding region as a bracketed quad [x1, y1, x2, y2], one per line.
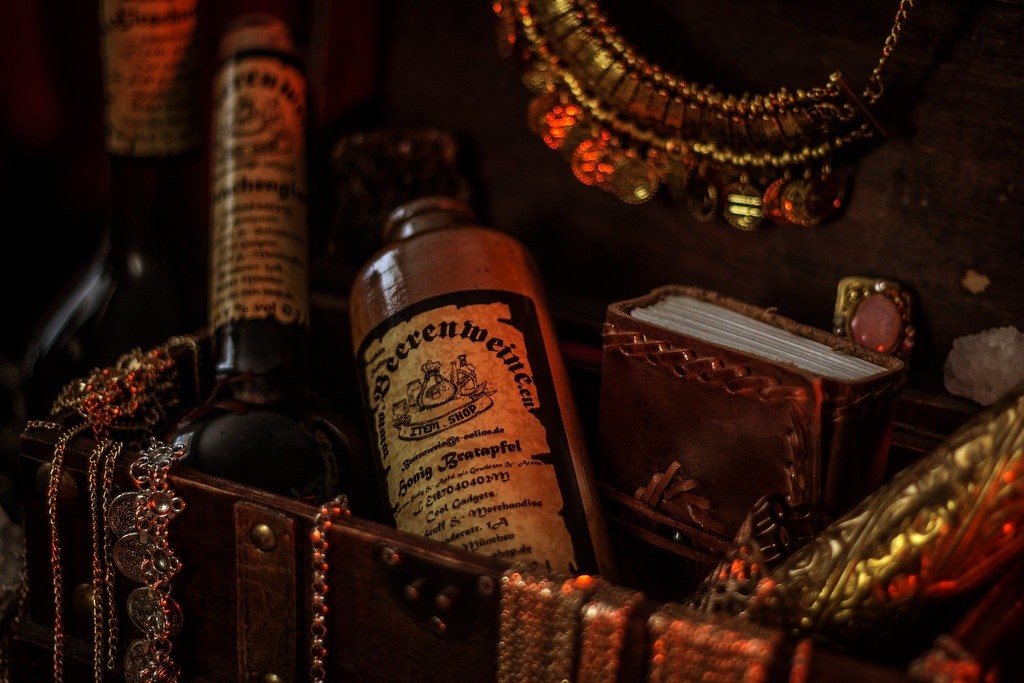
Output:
[495, 2, 914, 232]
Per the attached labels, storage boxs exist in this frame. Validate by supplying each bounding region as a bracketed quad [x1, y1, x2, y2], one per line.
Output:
[16, 333, 1024, 683]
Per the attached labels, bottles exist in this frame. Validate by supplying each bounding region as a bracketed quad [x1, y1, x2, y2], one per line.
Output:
[98, 0, 212, 161]
[202, 12, 316, 410]
[346, 195, 618, 603]
